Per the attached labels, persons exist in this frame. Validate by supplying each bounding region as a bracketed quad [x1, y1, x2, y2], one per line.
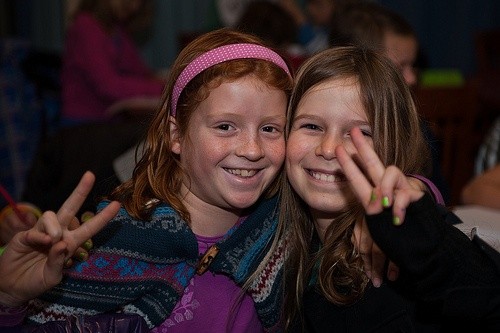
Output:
[1, 0, 342, 247]
[0, 25, 314, 332]
[61, 40, 500, 332]
[325, 5, 421, 97]
[458, 163, 500, 212]
